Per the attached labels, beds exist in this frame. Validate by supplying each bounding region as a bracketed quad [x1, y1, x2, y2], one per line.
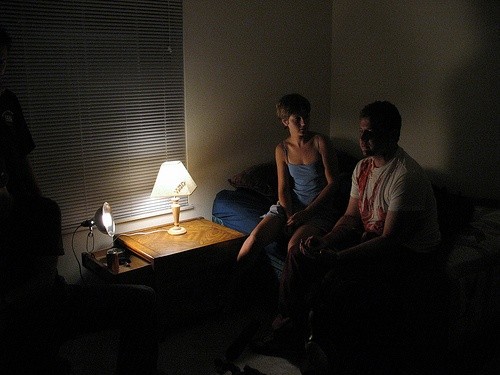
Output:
[213, 151, 500, 353]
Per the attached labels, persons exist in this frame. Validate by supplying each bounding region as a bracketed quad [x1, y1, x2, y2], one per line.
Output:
[0, 197, 79, 323]
[0, 23, 43, 214]
[251, 101, 442, 375]
[237, 93, 339, 275]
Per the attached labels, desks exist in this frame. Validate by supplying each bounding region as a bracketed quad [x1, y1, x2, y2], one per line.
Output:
[82, 215, 249, 303]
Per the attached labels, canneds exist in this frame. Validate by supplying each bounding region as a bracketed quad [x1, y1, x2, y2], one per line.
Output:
[106, 249, 119, 273]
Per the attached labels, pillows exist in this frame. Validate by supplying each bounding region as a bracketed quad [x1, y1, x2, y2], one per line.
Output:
[227, 160, 272, 198]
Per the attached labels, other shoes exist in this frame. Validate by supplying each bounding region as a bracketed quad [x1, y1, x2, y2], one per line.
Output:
[249, 329, 307, 363]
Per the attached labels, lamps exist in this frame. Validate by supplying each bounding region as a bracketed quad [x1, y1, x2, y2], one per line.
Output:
[152, 158, 197, 236]
[73, 201, 116, 301]
